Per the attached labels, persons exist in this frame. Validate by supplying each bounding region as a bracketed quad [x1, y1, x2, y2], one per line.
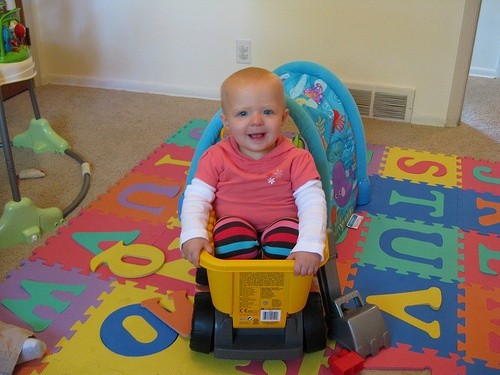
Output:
[178, 67, 328, 278]
